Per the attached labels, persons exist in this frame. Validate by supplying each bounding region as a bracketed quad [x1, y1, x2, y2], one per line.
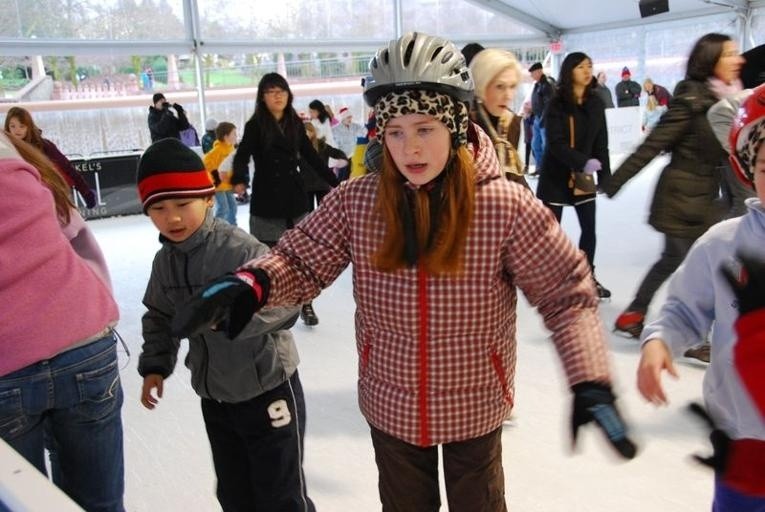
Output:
[600, 33, 744, 335]
[150, 91, 189, 145]
[135, 140, 314, 510]
[536, 51, 612, 304]
[4, 107, 96, 210]
[230, 72, 337, 324]
[596, 66, 671, 132]
[167, 32, 637, 512]
[1, 127, 125, 511]
[200, 119, 238, 225]
[305, 100, 366, 183]
[638, 83, 765, 512]
[463, 42, 556, 178]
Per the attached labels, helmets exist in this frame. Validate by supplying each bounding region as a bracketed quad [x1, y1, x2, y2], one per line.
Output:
[729, 83, 765, 190]
[361, 31, 474, 107]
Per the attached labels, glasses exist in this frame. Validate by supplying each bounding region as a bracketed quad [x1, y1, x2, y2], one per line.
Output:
[262, 90, 288, 97]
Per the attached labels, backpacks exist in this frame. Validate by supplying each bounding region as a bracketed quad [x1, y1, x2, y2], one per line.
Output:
[478, 105, 527, 185]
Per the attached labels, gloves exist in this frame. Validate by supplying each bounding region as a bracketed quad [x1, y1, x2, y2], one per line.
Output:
[720, 251, 765, 316]
[162, 102, 171, 110]
[191, 268, 271, 340]
[572, 382, 636, 459]
[584, 158, 601, 175]
[173, 103, 182, 112]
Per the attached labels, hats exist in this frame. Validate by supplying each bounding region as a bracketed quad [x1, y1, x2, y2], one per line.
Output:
[339, 107, 352, 121]
[529, 62, 542, 71]
[205, 119, 218, 131]
[137, 137, 215, 216]
[622, 67, 630, 77]
[153, 93, 164, 104]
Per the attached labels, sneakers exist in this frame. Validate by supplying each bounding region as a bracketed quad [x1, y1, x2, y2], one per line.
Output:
[616, 311, 644, 337]
[686, 339, 711, 362]
[301, 302, 318, 325]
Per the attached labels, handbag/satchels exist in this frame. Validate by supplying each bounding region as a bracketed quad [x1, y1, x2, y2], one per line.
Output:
[569, 172, 596, 196]
